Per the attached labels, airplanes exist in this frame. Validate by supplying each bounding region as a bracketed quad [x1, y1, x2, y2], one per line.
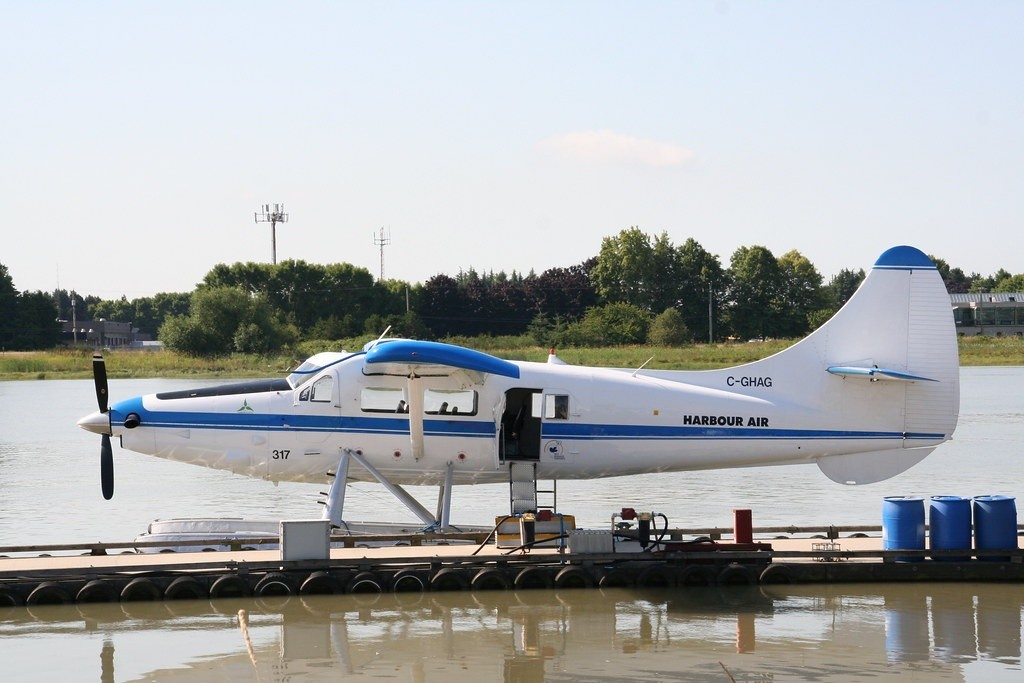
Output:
[79, 243, 961, 546]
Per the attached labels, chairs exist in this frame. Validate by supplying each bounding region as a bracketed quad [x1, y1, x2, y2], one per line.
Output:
[395, 398, 459, 415]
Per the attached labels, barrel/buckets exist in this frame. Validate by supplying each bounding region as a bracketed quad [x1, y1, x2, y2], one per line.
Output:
[973, 493, 1018, 562]
[929, 497, 971, 561]
[882, 494, 926, 562]
